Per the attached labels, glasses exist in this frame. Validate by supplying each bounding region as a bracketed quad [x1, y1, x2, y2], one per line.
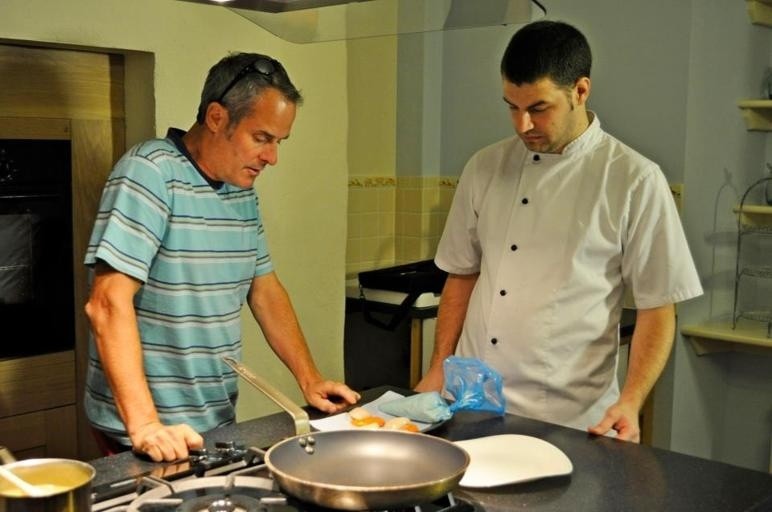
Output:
[217, 58, 286, 102]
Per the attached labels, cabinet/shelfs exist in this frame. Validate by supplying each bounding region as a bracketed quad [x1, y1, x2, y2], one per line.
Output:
[681, 0, 772, 355]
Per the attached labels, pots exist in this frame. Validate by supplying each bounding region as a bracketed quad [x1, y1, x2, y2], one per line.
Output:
[220, 355, 471, 510]
[0, 446, 96, 510]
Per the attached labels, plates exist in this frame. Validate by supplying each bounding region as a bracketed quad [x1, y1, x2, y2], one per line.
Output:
[311, 390, 456, 432]
[454, 434, 573, 490]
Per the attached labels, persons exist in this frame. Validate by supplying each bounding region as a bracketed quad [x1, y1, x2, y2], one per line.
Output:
[83, 53, 362, 463]
[413, 21, 703, 444]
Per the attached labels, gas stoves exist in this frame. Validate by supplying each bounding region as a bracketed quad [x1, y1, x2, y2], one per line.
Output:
[90, 439, 484, 512]
